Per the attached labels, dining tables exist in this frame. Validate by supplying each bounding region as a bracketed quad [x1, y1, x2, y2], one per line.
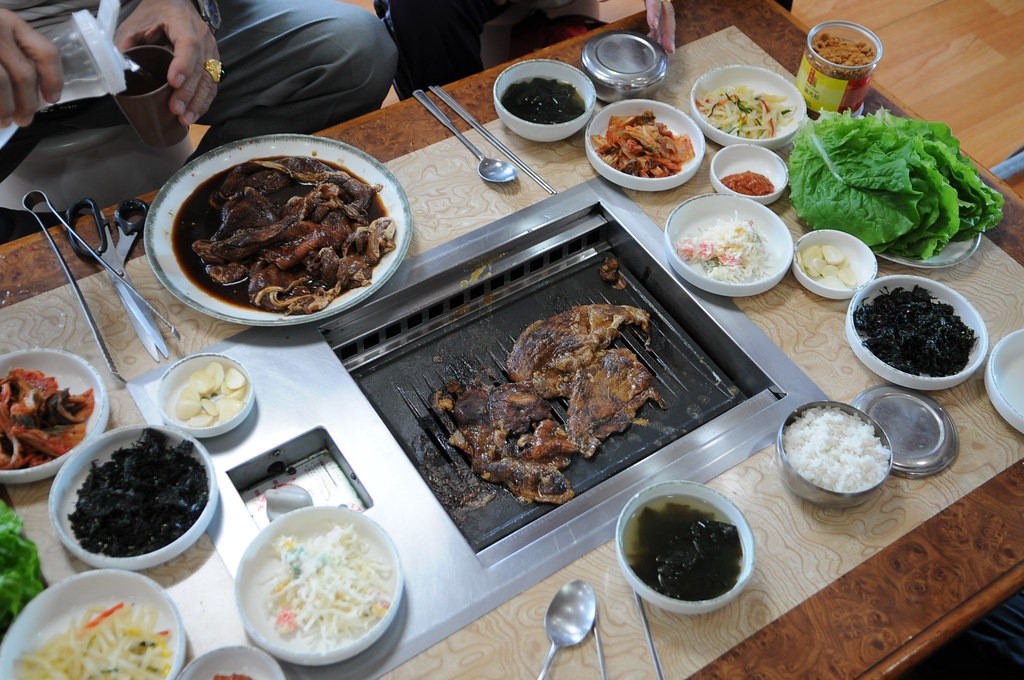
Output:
[0, 0, 1024, 680]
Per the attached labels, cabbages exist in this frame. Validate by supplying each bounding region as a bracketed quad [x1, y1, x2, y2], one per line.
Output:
[591, 110, 694, 178]
[0, 368, 94, 471]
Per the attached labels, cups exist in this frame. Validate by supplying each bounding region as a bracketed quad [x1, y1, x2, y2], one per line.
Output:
[113, 45, 189, 147]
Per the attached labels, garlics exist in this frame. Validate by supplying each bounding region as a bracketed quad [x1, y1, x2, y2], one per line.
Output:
[174, 361, 247, 428]
[796, 244, 857, 287]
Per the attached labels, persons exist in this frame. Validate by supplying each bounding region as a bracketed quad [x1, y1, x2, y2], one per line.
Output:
[0, 0, 400, 242]
[384, 0, 676, 102]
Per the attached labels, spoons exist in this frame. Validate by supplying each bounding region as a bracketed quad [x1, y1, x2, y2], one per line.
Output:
[536, 579, 596, 680]
[412, 90, 517, 182]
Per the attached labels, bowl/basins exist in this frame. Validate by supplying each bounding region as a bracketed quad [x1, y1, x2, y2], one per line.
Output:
[0, 348, 109, 484]
[691, 65, 807, 149]
[665, 194, 793, 296]
[792, 229, 879, 300]
[493, 58, 597, 141]
[48, 424, 218, 571]
[0, 569, 187, 680]
[234, 506, 403, 665]
[582, 31, 666, 101]
[615, 481, 755, 616]
[983, 328, 1024, 436]
[177, 645, 285, 680]
[157, 353, 254, 437]
[845, 274, 989, 390]
[709, 144, 789, 204]
[775, 401, 893, 508]
[584, 99, 706, 191]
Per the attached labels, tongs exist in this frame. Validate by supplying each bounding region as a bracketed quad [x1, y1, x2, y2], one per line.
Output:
[22, 190, 181, 383]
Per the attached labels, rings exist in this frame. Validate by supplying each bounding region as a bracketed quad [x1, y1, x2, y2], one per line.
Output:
[204, 58, 222, 82]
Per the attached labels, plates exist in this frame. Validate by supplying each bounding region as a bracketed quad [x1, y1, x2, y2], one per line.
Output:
[143, 133, 412, 326]
[875, 233, 981, 268]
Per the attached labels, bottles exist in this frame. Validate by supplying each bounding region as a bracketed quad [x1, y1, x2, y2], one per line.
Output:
[38, 0, 126, 110]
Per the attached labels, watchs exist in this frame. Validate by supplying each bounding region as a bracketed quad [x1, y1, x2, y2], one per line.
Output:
[198, 0, 221, 36]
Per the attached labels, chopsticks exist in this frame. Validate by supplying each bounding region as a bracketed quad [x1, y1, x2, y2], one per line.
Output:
[429, 85, 557, 194]
[592, 589, 665, 680]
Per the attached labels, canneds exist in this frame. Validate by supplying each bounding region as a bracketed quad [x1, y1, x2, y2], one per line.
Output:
[795, 21, 884, 118]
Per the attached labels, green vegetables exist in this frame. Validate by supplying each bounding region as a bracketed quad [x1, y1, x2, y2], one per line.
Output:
[789, 105, 1005, 261]
[0, 499, 45, 643]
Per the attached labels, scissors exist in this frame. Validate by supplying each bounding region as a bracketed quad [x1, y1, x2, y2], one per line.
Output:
[64, 197, 169, 363]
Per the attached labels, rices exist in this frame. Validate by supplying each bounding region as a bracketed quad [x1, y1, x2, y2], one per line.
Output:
[782, 406, 891, 492]
